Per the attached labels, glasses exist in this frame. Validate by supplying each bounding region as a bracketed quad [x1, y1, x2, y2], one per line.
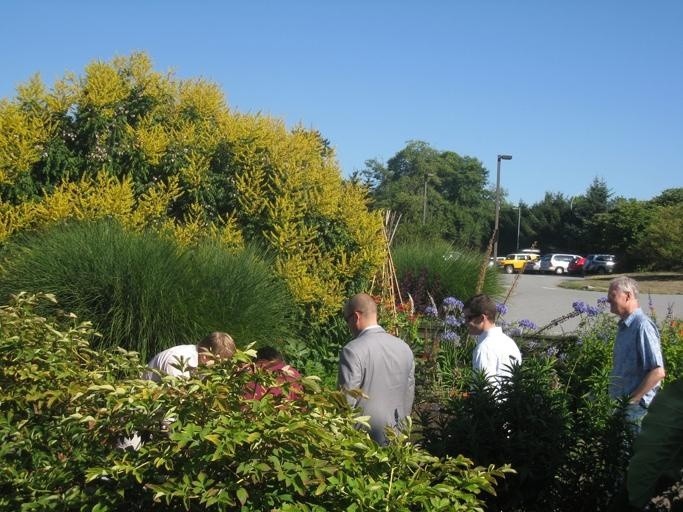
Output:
[464, 313, 487, 322]
[344, 311, 363, 324]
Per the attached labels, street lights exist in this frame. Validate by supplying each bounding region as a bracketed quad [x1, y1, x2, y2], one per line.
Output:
[421, 173, 434, 227]
[516, 205, 527, 249]
[494, 155, 512, 262]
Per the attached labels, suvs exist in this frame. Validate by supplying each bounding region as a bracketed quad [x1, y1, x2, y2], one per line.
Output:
[583, 254, 624, 274]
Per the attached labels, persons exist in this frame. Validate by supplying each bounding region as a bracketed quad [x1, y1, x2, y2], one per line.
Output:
[338, 293, 416, 450]
[461, 292, 522, 443]
[101, 331, 236, 482]
[603, 374, 683, 511]
[605, 275, 665, 435]
[237, 345, 307, 458]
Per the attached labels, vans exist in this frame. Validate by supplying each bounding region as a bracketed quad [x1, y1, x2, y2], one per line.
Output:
[541, 253, 582, 274]
[497, 253, 540, 274]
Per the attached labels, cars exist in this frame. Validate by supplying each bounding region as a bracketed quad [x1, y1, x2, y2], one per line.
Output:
[487, 256, 505, 269]
[442, 251, 472, 264]
[567, 257, 587, 274]
[523, 258, 541, 274]
